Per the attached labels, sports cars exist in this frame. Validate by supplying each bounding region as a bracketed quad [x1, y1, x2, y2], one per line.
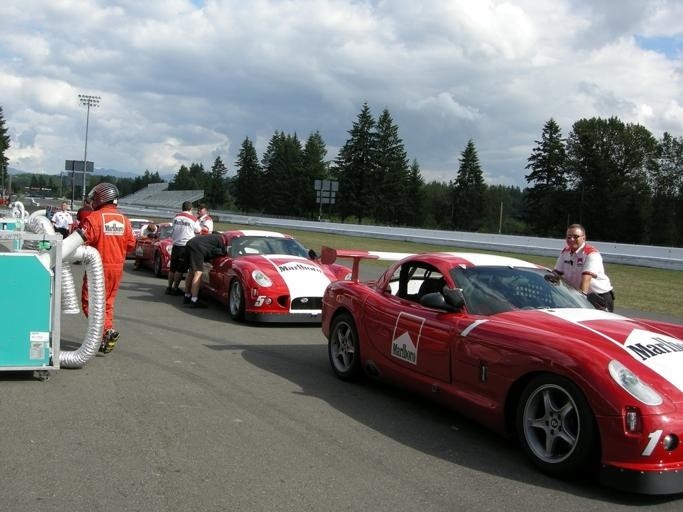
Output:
[312, 247, 683, 498]
[135, 223, 215, 275]
[125, 218, 156, 257]
[201, 228, 360, 327]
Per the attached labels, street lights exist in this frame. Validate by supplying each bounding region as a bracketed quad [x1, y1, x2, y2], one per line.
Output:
[77, 94, 102, 202]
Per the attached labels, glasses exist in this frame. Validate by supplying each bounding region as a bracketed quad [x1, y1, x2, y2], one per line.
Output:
[567, 235, 580, 239]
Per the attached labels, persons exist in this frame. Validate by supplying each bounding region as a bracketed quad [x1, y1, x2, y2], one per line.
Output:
[77, 198, 92, 220]
[50, 199, 73, 239]
[194, 203, 214, 237]
[182, 230, 227, 310]
[164, 198, 201, 297]
[78, 182, 137, 355]
[133, 221, 158, 271]
[553, 221, 615, 314]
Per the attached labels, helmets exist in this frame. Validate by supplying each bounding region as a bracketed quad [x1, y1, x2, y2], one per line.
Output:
[88, 183, 119, 210]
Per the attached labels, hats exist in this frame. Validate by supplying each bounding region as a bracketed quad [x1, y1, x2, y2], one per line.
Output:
[198, 203, 207, 208]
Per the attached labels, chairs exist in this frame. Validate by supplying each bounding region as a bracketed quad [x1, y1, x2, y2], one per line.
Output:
[418, 277, 446, 299]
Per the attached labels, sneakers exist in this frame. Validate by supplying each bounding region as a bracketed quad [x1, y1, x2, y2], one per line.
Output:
[99, 341, 105, 352]
[190, 300, 208, 308]
[184, 295, 191, 304]
[104, 329, 120, 353]
[171, 289, 185, 296]
[165, 287, 171, 294]
